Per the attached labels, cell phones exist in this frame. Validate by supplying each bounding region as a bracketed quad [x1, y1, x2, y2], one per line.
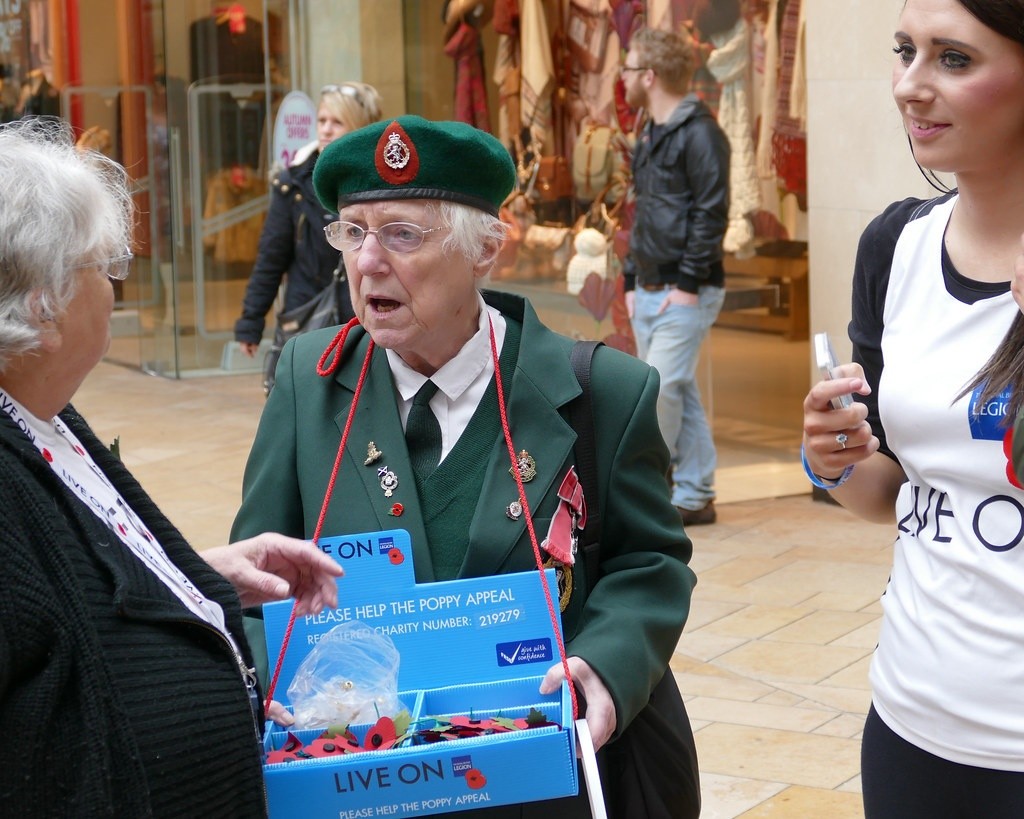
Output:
[815, 331, 854, 410]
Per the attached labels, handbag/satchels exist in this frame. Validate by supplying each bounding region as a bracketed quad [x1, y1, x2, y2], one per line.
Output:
[525, 220, 573, 279]
[567, 228, 616, 295]
[535, 155, 569, 202]
[264, 280, 340, 399]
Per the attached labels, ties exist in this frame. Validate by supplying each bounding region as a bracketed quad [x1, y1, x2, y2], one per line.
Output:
[403, 380, 442, 479]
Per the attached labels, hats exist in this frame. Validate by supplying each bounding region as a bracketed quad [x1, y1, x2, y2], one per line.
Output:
[312, 115, 516, 218]
[695, 0, 741, 43]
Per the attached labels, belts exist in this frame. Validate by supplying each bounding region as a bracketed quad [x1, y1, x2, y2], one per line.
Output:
[641, 282, 680, 291]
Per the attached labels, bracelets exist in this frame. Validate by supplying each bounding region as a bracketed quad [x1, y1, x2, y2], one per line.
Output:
[801, 444, 854, 489]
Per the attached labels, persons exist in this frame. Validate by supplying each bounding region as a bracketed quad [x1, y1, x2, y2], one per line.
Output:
[19, 69, 60, 140]
[617, 28, 732, 527]
[228, 115, 702, 818]
[950, 249, 1024, 484]
[800, 1, 1024, 819]
[0, 125, 344, 817]
[443, 0, 495, 132]
[233, 81, 384, 395]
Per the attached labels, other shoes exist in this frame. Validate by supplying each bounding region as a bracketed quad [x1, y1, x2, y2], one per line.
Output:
[673, 500, 717, 527]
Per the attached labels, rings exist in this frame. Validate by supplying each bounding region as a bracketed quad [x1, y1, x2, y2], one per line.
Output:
[836, 434, 847, 449]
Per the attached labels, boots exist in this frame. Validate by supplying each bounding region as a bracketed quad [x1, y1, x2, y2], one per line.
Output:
[501, 245, 534, 282]
[535, 246, 553, 282]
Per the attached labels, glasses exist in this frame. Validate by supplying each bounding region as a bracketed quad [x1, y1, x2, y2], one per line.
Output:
[620, 64, 659, 77]
[67, 243, 135, 280]
[320, 82, 363, 106]
[323, 221, 450, 254]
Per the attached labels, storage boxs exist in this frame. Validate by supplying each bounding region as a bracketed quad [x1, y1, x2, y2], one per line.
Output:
[261, 529, 581, 819]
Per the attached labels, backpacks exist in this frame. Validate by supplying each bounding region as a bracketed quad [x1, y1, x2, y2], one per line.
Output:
[572, 122, 626, 204]
